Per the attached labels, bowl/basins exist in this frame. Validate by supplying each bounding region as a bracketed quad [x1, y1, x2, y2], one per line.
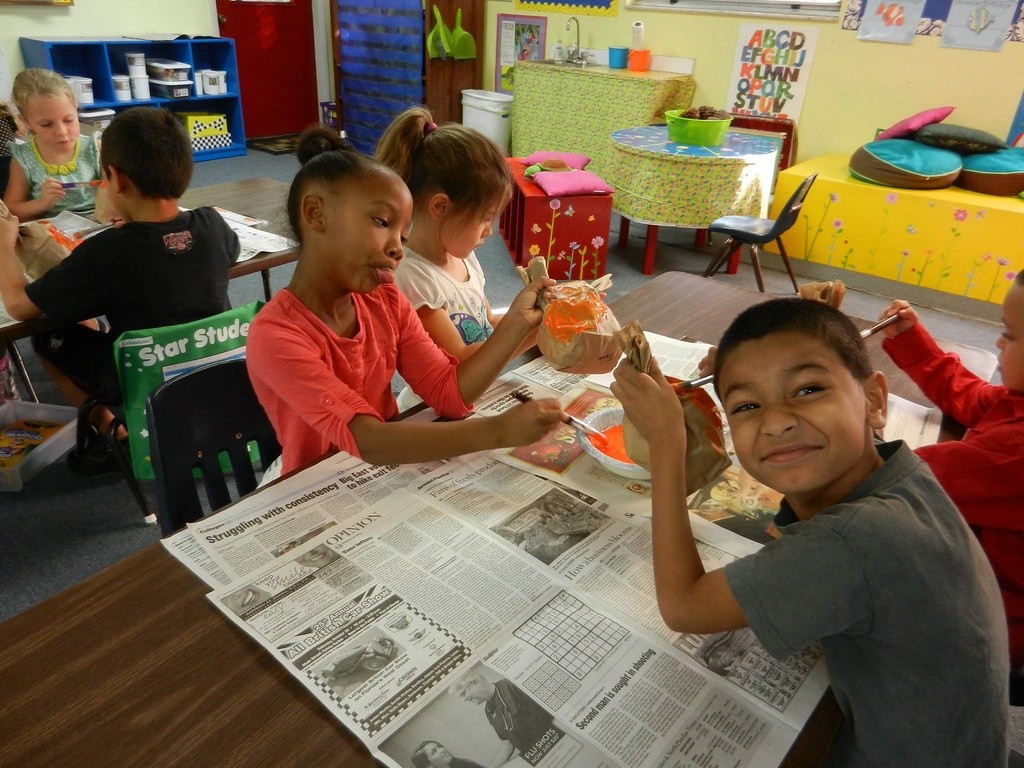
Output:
[664, 109, 734, 146]
[578, 404, 652, 481]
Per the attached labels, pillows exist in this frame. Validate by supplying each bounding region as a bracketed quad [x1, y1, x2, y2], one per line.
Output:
[849, 106, 1024, 198]
[530, 170, 615, 198]
[520, 150, 592, 171]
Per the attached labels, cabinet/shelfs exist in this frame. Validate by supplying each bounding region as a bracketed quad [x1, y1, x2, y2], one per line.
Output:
[20, 35, 248, 165]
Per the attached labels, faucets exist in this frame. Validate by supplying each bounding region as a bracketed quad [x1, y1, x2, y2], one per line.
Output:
[565, 17, 596, 66]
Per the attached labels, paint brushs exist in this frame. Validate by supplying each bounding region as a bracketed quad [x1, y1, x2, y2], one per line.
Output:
[73, 221, 115, 239]
[61, 179, 100, 188]
[670, 374, 714, 395]
[511, 391, 610, 445]
[859, 306, 913, 340]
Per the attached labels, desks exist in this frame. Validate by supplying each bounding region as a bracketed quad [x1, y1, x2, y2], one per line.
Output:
[510, 60, 698, 176]
[0, 261, 999, 768]
[498, 157, 613, 280]
[0, 208, 299, 473]
[608, 126, 778, 275]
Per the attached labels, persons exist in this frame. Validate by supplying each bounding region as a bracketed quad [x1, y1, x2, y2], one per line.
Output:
[610, 297, 1011, 768]
[878, 268, 1024, 707]
[458, 671, 554, 768]
[2, 68, 103, 223]
[0, 99, 19, 198]
[411, 740, 484, 768]
[221, 584, 271, 616]
[246, 126, 562, 476]
[0, 107, 240, 475]
[373, 107, 608, 413]
[294, 543, 340, 568]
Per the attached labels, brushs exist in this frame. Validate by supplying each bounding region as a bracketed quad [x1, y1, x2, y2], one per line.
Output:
[432, 4, 452, 59]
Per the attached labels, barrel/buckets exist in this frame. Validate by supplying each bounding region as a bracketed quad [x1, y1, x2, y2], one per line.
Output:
[608, 46, 629, 68]
[627, 49, 651, 71]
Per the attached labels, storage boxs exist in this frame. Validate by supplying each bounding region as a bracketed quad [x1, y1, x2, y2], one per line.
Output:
[149, 78, 194, 99]
[0, 400, 79, 492]
[178, 112, 231, 151]
[145, 59, 191, 82]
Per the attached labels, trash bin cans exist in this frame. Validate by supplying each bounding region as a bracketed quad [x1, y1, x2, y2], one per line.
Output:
[461, 88, 514, 156]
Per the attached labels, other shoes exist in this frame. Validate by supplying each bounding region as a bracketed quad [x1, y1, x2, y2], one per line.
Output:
[68, 437, 132, 473]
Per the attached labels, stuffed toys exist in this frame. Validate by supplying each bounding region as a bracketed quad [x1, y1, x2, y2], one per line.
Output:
[524, 158, 578, 179]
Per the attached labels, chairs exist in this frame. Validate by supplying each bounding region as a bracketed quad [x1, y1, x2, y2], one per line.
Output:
[81, 300, 261, 522]
[146, 360, 282, 539]
[701, 173, 820, 295]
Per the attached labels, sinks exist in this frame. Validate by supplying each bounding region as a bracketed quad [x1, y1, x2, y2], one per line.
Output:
[524, 59, 602, 68]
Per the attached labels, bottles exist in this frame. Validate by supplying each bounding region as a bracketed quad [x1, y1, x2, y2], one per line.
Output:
[194, 65, 228, 95]
[554, 39, 564, 61]
[64, 52, 150, 103]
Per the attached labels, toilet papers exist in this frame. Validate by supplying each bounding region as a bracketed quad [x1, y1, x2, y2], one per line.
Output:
[633, 21, 644, 50]
